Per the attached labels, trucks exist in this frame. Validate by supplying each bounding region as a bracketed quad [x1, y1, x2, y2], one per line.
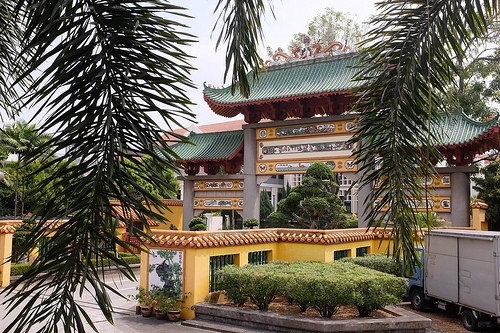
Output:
[403, 229, 500, 331]
